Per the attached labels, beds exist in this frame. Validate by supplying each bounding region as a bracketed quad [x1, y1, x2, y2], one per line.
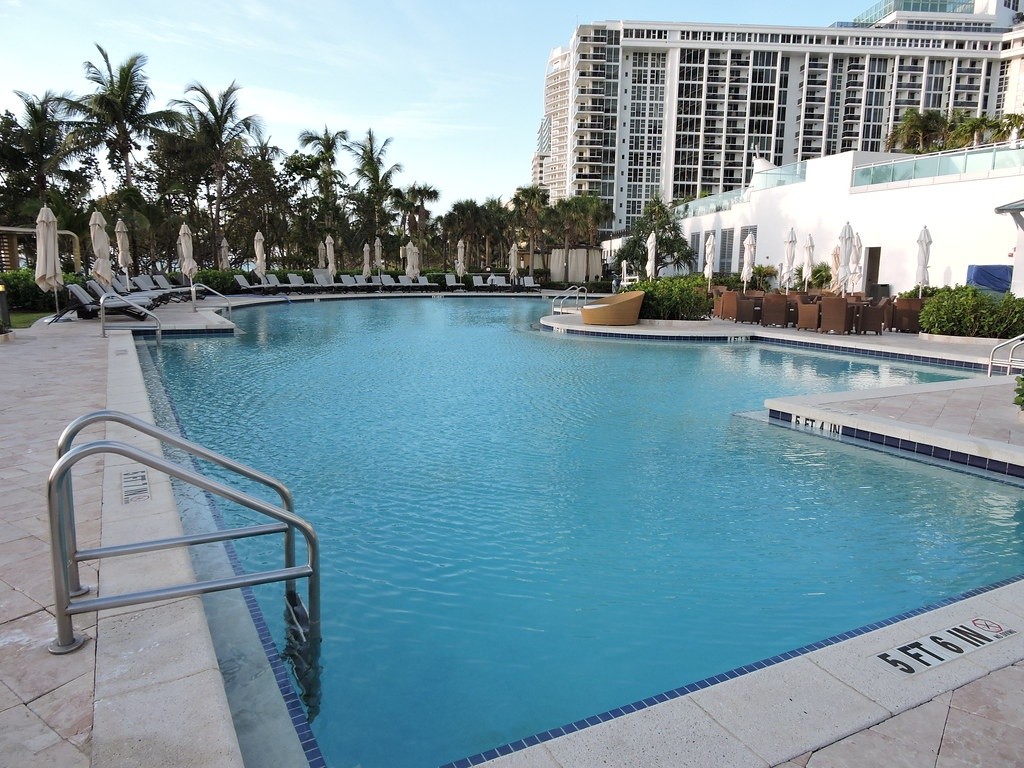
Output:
[581, 291, 645, 325]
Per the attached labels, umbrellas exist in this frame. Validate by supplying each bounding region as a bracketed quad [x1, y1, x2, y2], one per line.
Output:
[254, 230, 265, 284]
[457, 240, 465, 283]
[179, 222, 197, 287]
[35, 202, 64, 317]
[704, 233, 715, 293]
[176, 236, 185, 285]
[508, 243, 519, 284]
[406, 241, 419, 283]
[374, 237, 382, 282]
[318, 241, 326, 270]
[644, 231, 655, 283]
[89, 207, 112, 299]
[783, 228, 797, 294]
[363, 243, 371, 282]
[802, 234, 814, 292]
[915, 225, 932, 299]
[114, 218, 133, 291]
[832, 221, 861, 299]
[325, 233, 336, 281]
[221, 238, 229, 271]
[740, 231, 755, 294]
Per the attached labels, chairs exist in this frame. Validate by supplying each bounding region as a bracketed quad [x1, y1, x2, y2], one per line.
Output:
[712, 289, 924, 336]
[66, 274, 540, 321]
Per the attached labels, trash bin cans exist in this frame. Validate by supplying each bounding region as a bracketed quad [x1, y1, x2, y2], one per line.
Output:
[0, 279, 13, 334]
[869, 283, 889, 306]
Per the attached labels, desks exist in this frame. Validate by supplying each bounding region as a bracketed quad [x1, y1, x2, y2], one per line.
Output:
[847, 301, 870, 335]
